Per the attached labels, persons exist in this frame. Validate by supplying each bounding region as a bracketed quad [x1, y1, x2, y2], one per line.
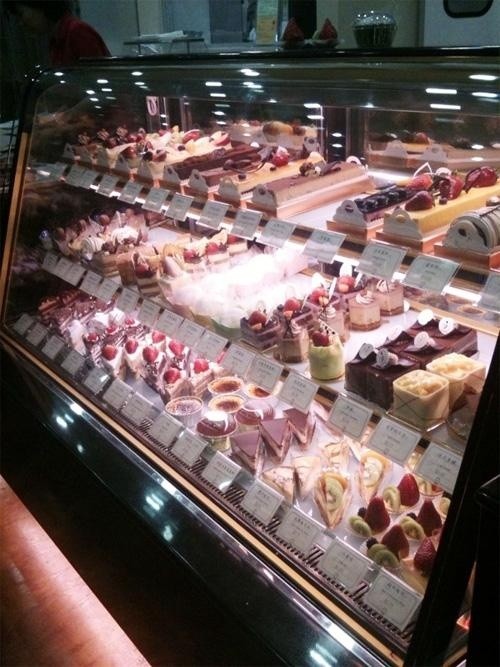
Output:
[10, 0, 144, 139]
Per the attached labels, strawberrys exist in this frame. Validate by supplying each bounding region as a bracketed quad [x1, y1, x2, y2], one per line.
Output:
[320, 18, 337, 40]
[282, 17, 305, 40]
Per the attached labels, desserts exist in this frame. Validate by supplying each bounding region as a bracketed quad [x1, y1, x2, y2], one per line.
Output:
[9, 118, 500, 598]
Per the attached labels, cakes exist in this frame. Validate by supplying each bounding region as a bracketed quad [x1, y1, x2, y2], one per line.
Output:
[352, 14, 396, 50]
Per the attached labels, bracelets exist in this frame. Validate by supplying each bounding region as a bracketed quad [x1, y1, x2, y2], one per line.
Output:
[48, 112, 61, 126]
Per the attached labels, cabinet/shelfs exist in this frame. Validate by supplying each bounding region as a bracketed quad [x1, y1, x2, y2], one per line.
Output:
[1, 45, 500, 667]
